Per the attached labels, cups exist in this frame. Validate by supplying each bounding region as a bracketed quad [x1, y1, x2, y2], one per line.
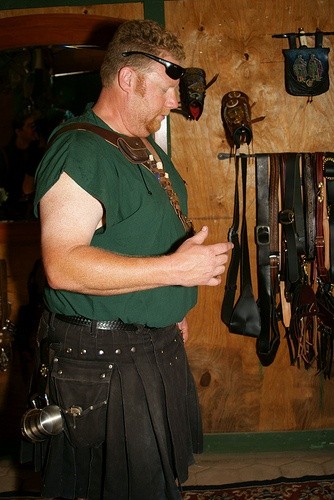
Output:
[19, 393, 64, 443]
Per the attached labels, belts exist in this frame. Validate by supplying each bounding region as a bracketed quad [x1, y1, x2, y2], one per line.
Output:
[46, 309, 136, 332]
[221, 151, 334, 379]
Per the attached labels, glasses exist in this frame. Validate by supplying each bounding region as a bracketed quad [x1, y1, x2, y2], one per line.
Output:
[123, 51, 185, 79]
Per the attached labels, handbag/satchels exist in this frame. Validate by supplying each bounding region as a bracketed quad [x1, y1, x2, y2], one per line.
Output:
[281, 30, 330, 103]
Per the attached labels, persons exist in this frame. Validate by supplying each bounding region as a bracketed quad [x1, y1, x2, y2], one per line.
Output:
[21, 20, 234, 500]
